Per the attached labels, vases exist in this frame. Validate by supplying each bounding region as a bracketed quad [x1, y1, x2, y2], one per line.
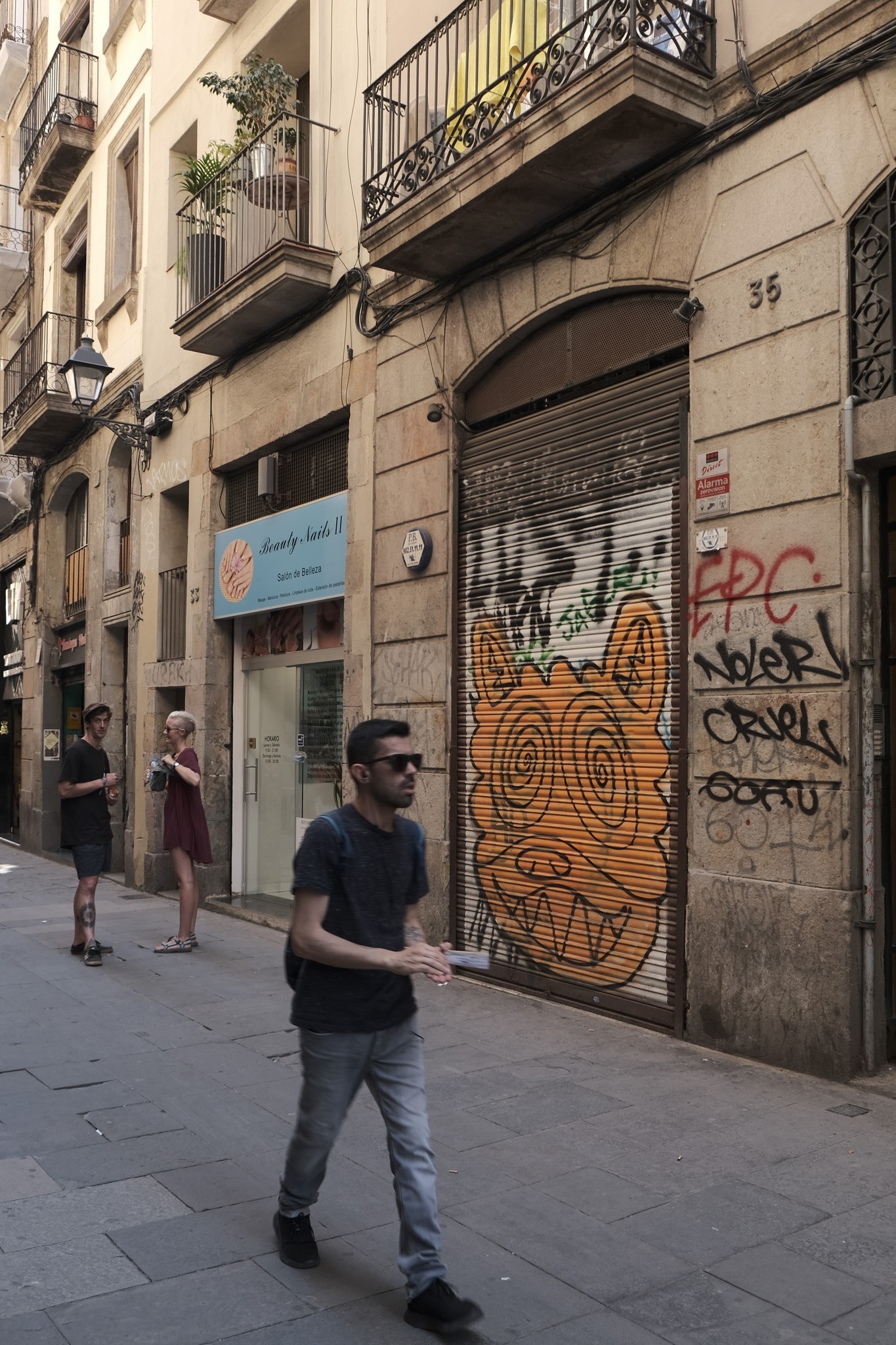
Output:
[58, 114, 72, 125]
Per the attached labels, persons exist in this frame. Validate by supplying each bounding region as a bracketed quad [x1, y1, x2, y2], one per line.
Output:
[150, 712, 214, 954]
[271, 718, 485, 1332]
[60, 702, 119, 966]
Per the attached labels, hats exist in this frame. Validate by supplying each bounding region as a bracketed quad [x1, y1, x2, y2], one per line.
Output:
[82, 702, 110, 721]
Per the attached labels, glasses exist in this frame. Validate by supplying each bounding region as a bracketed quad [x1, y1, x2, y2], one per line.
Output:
[165, 726, 181, 733]
[371, 753, 423, 772]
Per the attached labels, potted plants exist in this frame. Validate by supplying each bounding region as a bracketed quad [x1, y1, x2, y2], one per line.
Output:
[166, 47, 306, 308]
[74, 97, 96, 130]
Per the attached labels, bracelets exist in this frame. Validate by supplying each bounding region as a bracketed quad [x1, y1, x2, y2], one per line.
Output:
[101, 776, 107, 786]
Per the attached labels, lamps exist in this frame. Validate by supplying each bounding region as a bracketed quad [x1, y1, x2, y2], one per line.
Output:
[672, 297, 704, 323]
[57, 334, 151, 459]
[427, 404, 443, 422]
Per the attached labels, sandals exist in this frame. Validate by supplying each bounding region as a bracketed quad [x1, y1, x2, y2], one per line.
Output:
[154, 935, 192, 953]
[167, 936, 198, 947]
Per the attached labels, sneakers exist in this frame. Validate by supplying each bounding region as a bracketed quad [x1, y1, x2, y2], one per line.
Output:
[71, 939, 113, 953]
[84, 946, 102, 965]
[404, 1277, 484, 1332]
[273, 1210, 321, 1268]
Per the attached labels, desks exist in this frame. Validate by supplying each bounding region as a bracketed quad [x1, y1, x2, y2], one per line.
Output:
[244, 173, 310, 250]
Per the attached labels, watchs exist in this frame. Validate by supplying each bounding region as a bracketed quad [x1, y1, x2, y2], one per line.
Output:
[172, 762, 180, 769]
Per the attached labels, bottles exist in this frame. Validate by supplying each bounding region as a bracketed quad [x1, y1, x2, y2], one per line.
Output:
[151, 758, 158, 781]
[108, 783, 117, 799]
